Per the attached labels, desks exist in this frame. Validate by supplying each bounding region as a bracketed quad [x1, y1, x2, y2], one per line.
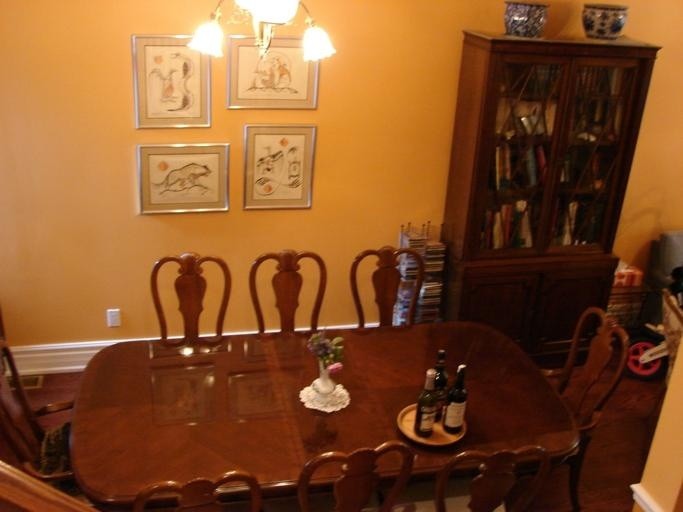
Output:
[607, 280, 657, 356]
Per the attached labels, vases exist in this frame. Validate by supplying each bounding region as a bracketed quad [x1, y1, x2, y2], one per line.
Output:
[297, 357, 356, 416]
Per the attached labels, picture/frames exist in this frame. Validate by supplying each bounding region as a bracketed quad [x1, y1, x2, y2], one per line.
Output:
[241, 125, 320, 212]
[226, 371, 294, 419]
[130, 35, 213, 131]
[241, 334, 312, 363]
[149, 372, 217, 429]
[133, 144, 232, 215]
[224, 36, 320, 113]
[147, 332, 235, 359]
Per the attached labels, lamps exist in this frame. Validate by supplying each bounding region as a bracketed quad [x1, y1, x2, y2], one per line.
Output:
[186, 0, 336, 65]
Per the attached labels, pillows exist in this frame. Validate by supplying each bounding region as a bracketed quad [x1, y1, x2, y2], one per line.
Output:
[659, 230, 682, 273]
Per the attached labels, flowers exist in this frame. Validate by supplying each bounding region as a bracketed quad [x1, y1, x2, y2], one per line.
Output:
[303, 329, 354, 375]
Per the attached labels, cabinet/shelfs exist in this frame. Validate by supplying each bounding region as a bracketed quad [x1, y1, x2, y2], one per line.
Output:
[442, 28, 663, 262]
[440, 251, 620, 379]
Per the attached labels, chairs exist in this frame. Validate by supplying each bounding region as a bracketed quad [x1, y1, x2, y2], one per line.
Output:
[247, 249, 327, 333]
[507, 308, 631, 511]
[131, 467, 266, 512]
[296, 442, 414, 512]
[644, 238, 682, 341]
[151, 252, 234, 340]
[0, 324, 76, 484]
[349, 246, 425, 327]
[435, 447, 548, 511]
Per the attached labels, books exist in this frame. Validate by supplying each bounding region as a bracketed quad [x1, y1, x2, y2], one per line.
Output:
[399, 223, 444, 322]
[476, 60, 632, 251]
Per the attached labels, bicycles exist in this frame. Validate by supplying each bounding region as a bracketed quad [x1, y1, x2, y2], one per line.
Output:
[625, 267, 683, 382]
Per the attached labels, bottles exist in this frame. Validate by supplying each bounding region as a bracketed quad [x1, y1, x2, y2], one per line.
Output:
[413, 369, 438, 437]
[442, 362, 469, 431]
[433, 349, 450, 422]
